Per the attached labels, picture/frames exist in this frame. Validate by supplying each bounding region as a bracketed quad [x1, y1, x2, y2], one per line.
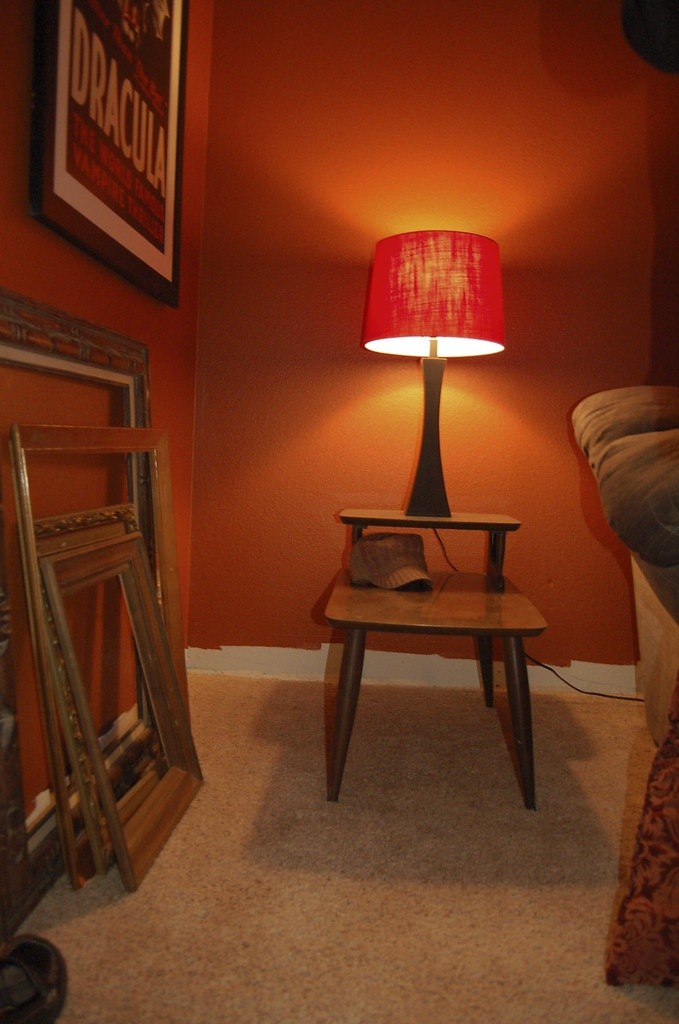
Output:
[28, 0, 189, 308]
[0, 285, 205, 942]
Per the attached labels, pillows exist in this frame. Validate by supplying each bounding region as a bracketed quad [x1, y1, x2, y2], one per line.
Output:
[607, 687, 679, 996]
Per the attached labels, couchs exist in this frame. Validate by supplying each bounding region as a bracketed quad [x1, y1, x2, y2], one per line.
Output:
[571, 385, 679, 746]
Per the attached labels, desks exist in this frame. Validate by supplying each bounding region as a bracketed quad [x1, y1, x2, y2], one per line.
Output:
[325, 508, 548, 812]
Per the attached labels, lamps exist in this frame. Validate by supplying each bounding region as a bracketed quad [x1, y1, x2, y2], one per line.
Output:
[361, 230, 506, 518]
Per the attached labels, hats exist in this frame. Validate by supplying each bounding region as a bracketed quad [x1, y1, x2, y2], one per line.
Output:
[349, 533, 432, 589]
